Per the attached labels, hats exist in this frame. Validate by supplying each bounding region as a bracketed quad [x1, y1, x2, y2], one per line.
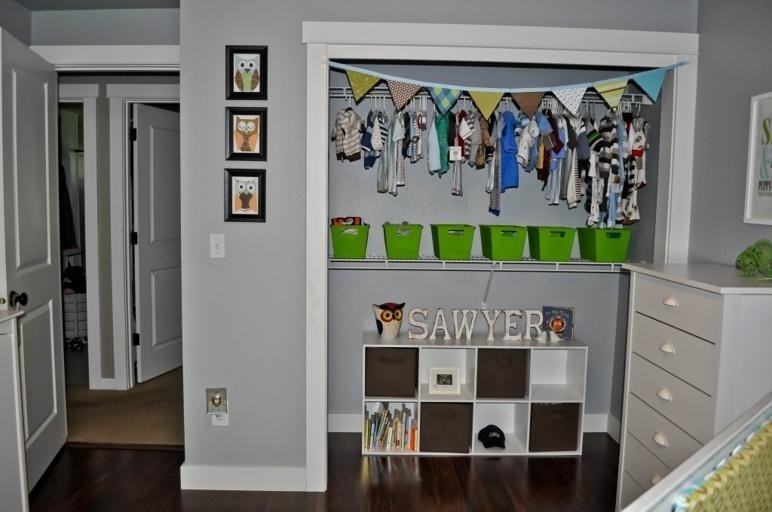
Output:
[478, 425, 505, 449]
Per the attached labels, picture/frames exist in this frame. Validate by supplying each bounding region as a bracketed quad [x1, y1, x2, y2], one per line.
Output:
[220, 43, 269, 223]
[426, 366, 461, 395]
[742, 91, 771, 227]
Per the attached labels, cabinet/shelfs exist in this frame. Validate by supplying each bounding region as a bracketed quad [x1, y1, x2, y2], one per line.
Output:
[357, 333, 589, 457]
[613, 263, 772, 510]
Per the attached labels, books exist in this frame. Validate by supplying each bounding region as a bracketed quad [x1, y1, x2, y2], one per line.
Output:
[363, 402, 417, 454]
[361, 454, 420, 490]
